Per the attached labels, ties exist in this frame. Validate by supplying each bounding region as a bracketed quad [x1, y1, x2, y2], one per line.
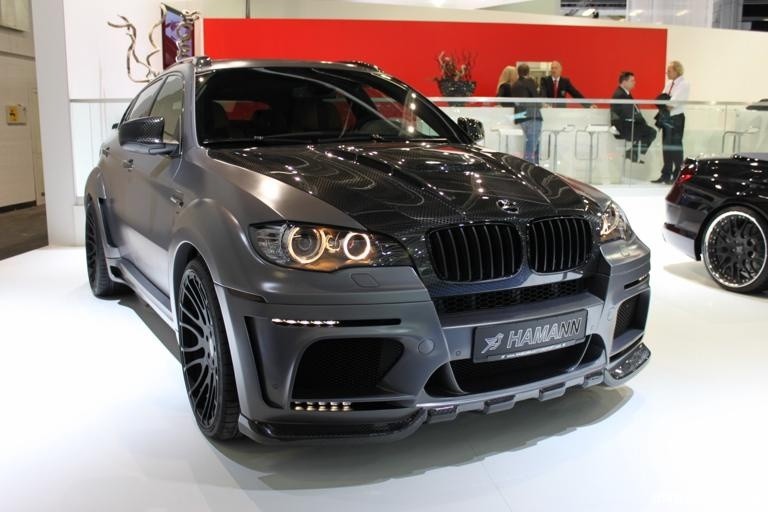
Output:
[554, 80, 557, 98]
[668, 82, 673, 95]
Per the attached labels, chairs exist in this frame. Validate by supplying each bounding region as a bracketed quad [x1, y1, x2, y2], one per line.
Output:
[209, 95, 357, 140]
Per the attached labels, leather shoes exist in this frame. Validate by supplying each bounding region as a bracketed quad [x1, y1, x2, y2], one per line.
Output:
[626, 149, 643, 164]
[652, 174, 676, 184]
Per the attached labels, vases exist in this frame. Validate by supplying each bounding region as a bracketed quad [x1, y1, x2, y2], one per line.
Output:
[437, 79, 477, 106]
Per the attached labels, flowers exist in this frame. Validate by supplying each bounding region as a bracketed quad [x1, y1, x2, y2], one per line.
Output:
[433, 47, 475, 80]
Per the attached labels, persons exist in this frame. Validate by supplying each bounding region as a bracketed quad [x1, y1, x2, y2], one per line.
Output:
[539, 61, 598, 108]
[651, 61, 688, 186]
[512, 64, 548, 165]
[612, 72, 657, 164]
[497, 66, 519, 108]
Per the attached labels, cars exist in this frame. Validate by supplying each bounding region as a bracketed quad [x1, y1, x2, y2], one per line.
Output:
[661, 152, 767, 294]
[84, 56, 652, 448]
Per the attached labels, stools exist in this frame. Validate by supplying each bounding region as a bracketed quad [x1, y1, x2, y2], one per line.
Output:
[490, 122, 639, 184]
[720, 116, 761, 158]
